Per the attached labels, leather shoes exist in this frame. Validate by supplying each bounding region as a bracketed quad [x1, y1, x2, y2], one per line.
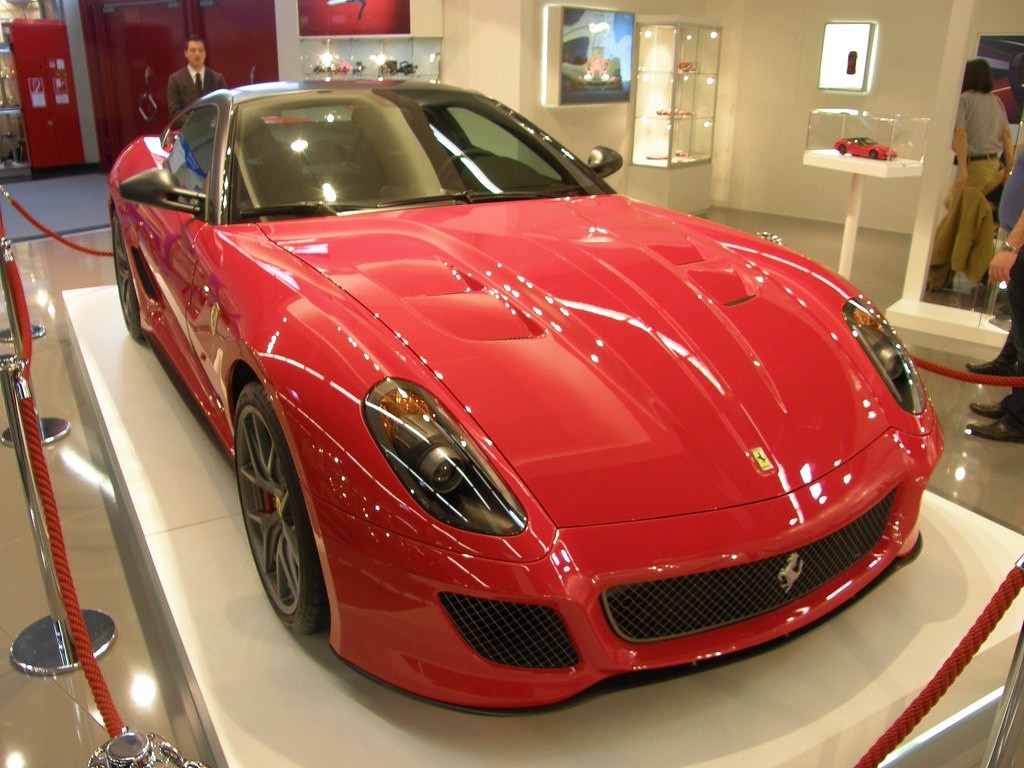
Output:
[967, 360, 1017, 376]
[966, 419, 1024, 441]
[970, 402, 1009, 419]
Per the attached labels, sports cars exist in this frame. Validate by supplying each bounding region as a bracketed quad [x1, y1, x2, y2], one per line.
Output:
[835, 135, 897, 160]
[106, 80, 948, 707]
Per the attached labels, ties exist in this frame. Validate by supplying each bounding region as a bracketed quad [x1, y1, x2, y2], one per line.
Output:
[195, 73, 202, 97]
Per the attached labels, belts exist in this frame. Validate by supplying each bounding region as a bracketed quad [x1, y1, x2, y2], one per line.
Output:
[970, 152, 996, 160]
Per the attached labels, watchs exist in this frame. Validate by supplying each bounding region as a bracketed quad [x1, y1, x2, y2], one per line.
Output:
[1002, 242, 1019, 254]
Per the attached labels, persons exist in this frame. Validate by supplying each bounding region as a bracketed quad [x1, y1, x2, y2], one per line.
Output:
[967, 147, 1024, 443]
[941, 60, 1014, 291]
[167, 35, 229, 129]
